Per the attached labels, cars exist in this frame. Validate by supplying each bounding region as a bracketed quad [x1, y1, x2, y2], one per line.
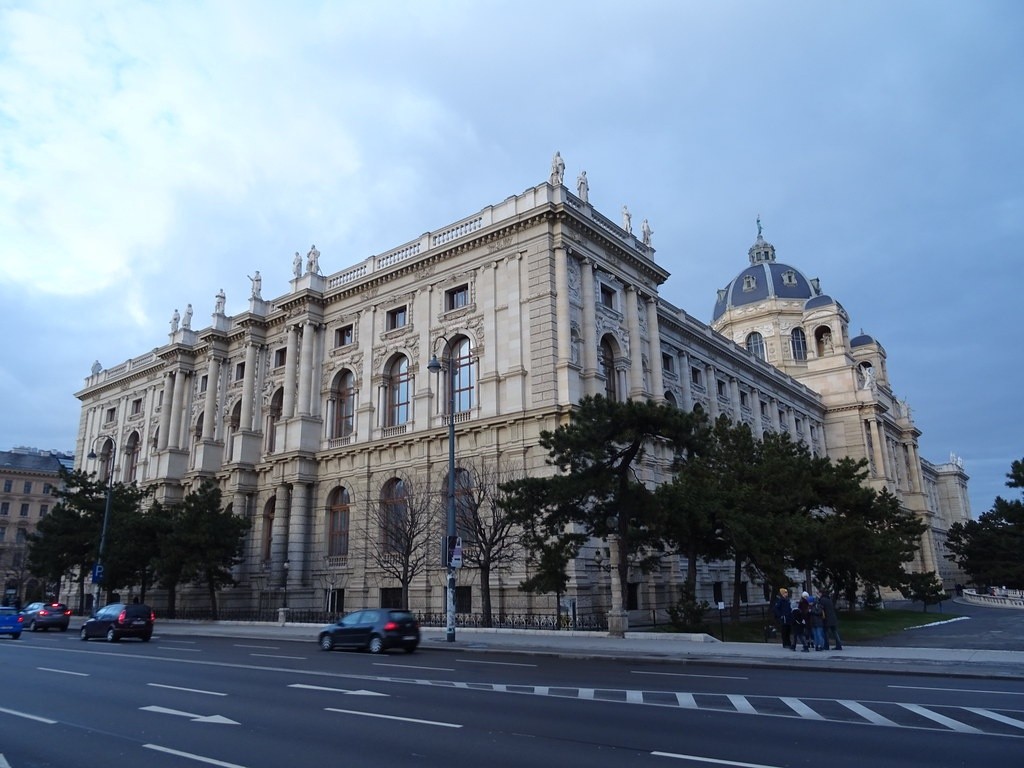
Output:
[79, 602, 155, 644]
[1, 606, 24, 639]
[319, 607, 422, 656]
[18, 602, 71, 632]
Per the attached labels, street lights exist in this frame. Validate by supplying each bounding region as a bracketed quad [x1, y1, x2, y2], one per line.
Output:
[428, 335, 459, 644]
[87, 435, 117, 619]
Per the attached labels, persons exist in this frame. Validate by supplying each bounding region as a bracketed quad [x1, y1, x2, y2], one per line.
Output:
[774, 587, 843, 653]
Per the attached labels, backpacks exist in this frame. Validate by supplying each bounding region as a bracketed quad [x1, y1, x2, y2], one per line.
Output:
[821, 610, 826, 619]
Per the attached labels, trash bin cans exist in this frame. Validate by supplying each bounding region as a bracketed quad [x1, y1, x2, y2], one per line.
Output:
[764, 625, 777, 639]
[277, 608, 290, 622]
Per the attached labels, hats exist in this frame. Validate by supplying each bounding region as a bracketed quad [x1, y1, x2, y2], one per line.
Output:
[807, 596, 814, 603]
[780, 588, 787, 595]
[802, 591, 809, 597]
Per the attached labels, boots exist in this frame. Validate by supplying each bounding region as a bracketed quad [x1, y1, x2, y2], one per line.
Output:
[801, 645, 809, 652]
